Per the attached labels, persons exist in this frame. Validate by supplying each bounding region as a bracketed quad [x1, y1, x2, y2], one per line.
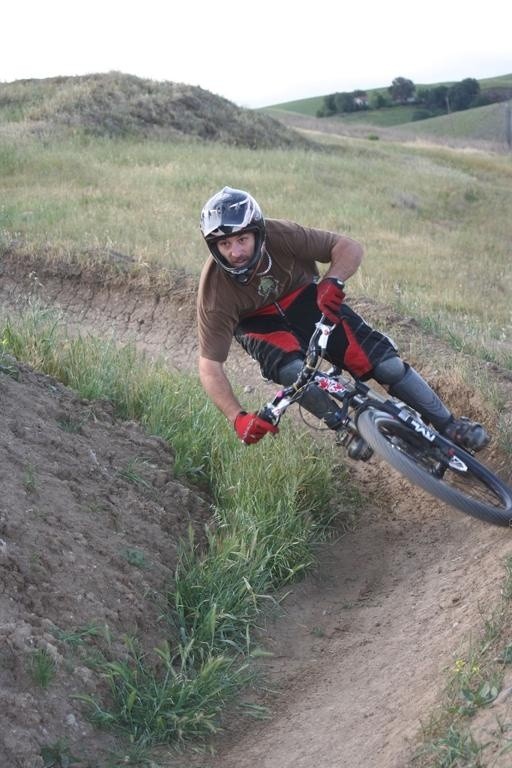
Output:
[192, 185, 490, 462]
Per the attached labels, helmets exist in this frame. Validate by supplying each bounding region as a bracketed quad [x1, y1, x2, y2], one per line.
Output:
[198, 185, 266, 280]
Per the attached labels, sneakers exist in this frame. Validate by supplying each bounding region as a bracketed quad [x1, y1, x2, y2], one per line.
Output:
[335, 424, 372, 464]
[445, 418, 489, 449]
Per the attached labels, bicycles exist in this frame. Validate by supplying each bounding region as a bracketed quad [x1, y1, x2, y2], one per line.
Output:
[250, 276, 512, 530]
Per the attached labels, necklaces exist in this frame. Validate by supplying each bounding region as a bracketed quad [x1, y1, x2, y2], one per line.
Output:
[257, 249, 272, 277]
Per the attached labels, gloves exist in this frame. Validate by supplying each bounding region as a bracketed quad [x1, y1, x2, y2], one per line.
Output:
[316, 276, 346, 323]
[230, 410, 281, 445]
